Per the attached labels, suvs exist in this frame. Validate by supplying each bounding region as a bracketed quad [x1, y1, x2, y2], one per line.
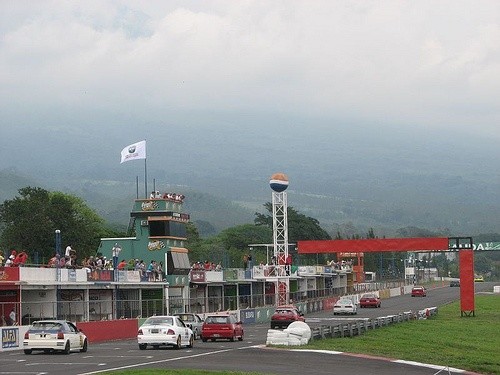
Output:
[270, 304, 306, 330]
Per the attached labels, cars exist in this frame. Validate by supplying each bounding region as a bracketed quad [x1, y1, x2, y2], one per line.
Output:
[21, 319, 89, 356]
[359, 293, 382, 309]
[411, 285, 428, 297]
[449, 280, 460, 287]
[200, 311, 245, 343]
[172, 312, 205, 341]
[333, 298, 358, 316]
[136, 315, 195, 350]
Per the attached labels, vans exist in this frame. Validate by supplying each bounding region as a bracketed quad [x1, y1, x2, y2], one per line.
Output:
[474, 276, 485, 283]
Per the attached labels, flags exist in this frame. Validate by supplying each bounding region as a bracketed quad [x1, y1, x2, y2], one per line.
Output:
[120, 141, 146, 163]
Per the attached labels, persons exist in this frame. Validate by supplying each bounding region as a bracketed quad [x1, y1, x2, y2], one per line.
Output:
[0, 242, 168, 282]
[242, 250, 291, 276]
[0, 307, 16, 327]
[193, 260, 221, 271]
[149, 191, 185, 201]
[327, 258, 351, 271]
